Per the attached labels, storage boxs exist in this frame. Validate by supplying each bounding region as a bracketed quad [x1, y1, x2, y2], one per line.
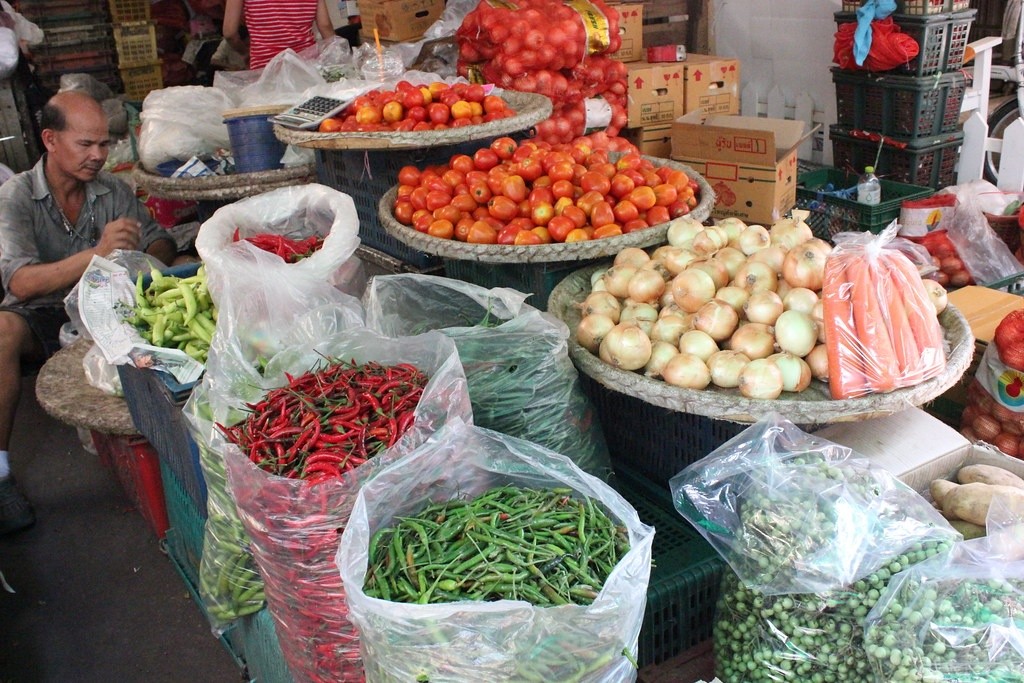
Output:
[87, 262, 294, 683]
[108, 0, 166, 160]
[354, 0, 1024, 671]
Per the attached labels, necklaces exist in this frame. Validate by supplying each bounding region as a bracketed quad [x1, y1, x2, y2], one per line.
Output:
[43, 178, 97, 241]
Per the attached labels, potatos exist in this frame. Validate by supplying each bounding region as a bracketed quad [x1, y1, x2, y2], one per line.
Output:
[927, 463, 1024, 540]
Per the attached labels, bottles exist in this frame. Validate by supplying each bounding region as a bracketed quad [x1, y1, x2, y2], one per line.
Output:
[857, 166, 881, 205]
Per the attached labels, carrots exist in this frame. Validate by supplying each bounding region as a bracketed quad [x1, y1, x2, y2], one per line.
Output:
[825, 244, 946, 397]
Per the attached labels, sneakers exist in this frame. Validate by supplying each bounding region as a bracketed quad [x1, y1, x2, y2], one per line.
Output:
[0, 472, 37, 533]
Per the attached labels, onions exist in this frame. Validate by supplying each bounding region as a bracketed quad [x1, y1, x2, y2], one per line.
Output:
[454, 0, 629, 142]
[959, 316, 1024, 464]
[576, 215, 832, 401]
[917, 231, 972, 285]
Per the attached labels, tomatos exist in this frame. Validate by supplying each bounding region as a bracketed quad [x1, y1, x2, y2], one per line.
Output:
[319, 80, 517, 132]
[394, 134, 697, 243]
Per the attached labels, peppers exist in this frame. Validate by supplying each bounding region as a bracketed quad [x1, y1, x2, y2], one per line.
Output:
[233, 227, 329, 262]
[188, 299, 640, 683]
[118, 257, 221, 367]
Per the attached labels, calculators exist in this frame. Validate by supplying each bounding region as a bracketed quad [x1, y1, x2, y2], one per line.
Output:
[266, 94, 353, 129]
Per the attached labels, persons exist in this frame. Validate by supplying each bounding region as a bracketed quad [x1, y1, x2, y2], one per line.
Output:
[222, 0, 334, 70]
[0, 91, 178, 531]
[0, 0, 45, 75]
